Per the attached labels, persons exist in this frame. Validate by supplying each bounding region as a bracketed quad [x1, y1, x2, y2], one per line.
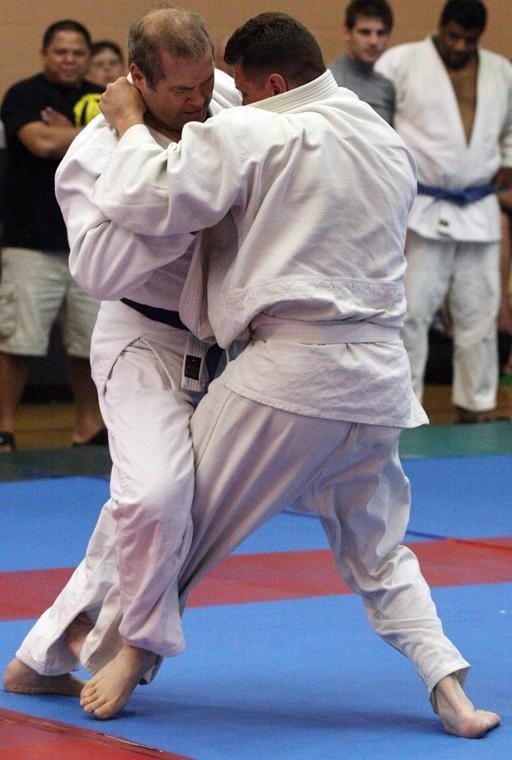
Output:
[326, 0, 396, 132]
[59, 11, 502, 739]
[0, 16, 109, 456]
[85, 39, 124, 89]
[371, 0, 511, 425]
[3, 4, 247, 725]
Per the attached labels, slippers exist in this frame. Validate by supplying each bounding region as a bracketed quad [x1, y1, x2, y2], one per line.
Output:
[0, 431, 15, 452]
[72, 427, 108, 446]
